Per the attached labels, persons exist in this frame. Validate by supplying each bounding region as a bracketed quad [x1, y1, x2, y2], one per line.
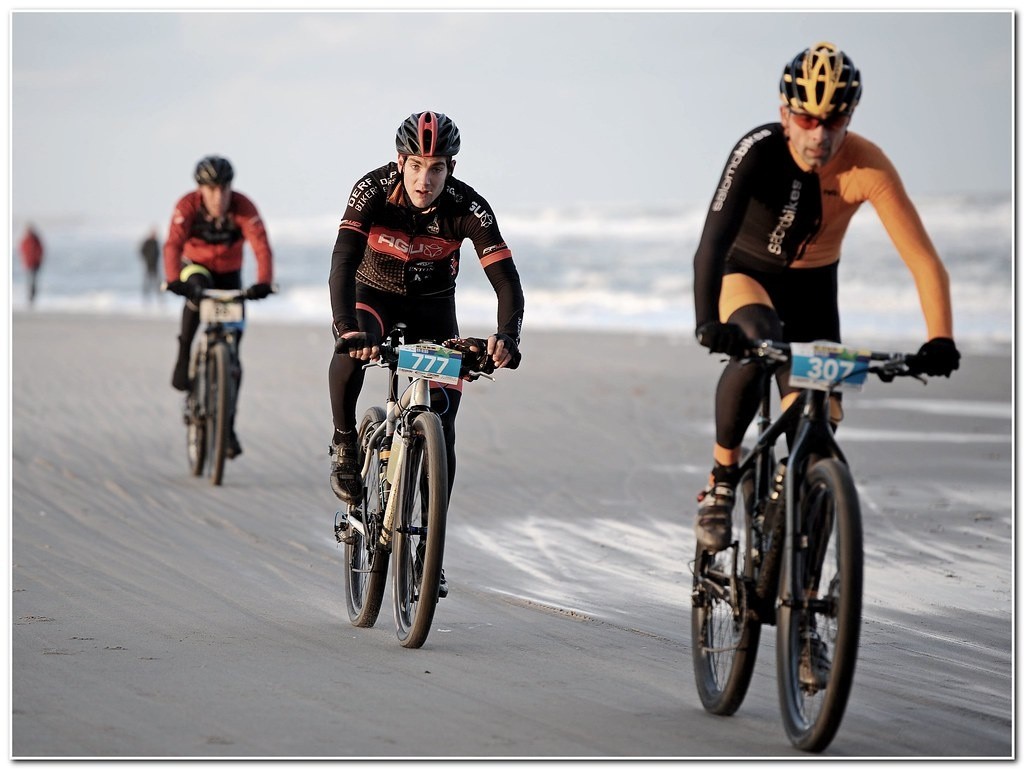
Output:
[139, 231, 161, 304]
[162, 156, 273, 457]
[19, 226, 42, 306]
[693, 41, 962, 691]
[328, 111, 524, 597]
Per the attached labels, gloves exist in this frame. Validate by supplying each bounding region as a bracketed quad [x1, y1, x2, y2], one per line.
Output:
[697, 320, 753, 363]
[909, 338, 960, 378]
[247, 283, 274, 301]
[166, 280, 195, 299]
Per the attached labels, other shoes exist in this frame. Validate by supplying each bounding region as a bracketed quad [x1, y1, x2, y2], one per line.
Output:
[173, 361, 190, 390]
[224, 429, 241, 458]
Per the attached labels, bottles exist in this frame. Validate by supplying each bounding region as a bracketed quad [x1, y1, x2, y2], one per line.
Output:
[380, 445, 390, 503]
[386, 423, 401, 485]
[762, 457, 788, 534]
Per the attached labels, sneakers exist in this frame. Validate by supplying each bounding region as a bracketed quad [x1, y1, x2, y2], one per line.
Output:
[695, 482, 736, 549]
[797, 625, 832, 689]
[414, 558, 449, 598]
[328, 438, 364, 505]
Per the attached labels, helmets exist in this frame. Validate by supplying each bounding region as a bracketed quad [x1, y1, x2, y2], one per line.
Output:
[780, 40, 862, 117]
[396, 111, 460, 157]
[195, 157, 234, 185]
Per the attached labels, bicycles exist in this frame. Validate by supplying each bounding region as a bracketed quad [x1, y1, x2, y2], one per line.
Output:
[687, 316, 929, 753]
[332, 319, 496, 651]
[162, 281, 278, 486]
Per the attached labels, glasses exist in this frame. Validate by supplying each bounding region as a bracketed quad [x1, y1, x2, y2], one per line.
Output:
[789, 104, 853, 131]
